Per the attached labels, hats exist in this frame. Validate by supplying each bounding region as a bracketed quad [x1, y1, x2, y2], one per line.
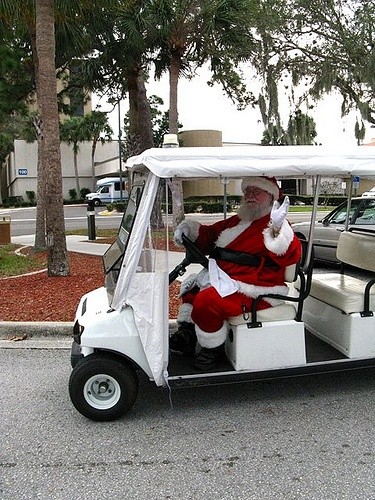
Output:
[241, 176, 283, 201]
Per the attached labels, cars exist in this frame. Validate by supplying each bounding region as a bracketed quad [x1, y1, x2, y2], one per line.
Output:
[289, 196, 375, 275]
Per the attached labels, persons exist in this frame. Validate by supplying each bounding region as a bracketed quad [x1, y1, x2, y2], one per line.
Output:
[169, 175, 301, 371]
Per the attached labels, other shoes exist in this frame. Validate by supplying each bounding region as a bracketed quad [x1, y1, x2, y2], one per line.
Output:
[191, 347, 226, 371]
[170, 326, 196, 356]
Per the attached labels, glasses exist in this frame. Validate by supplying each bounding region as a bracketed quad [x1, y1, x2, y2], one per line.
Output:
[243, 189, 263, 195]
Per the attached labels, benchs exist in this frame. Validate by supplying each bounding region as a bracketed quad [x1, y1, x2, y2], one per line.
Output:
[225, 233, 307, 326]
[291, 229, 375, 314]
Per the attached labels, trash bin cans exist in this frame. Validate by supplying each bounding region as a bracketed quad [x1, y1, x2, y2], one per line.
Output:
[0, 215, 11, 244]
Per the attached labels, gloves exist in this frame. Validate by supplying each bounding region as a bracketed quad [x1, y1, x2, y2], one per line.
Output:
[172, 225, 190, 247]
[271, 196, 290, 226]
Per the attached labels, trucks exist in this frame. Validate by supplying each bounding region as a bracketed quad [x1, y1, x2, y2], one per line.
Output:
[85, 176, 130, 206]
